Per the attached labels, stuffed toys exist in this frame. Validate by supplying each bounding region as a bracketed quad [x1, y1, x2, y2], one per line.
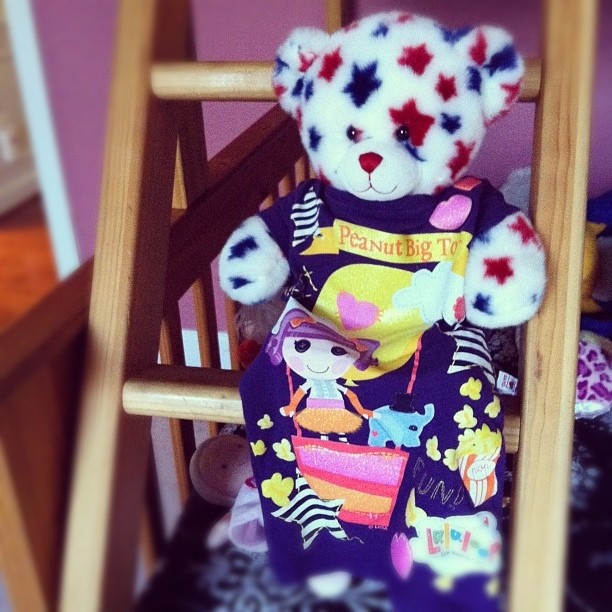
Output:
[190, 434, 271, 551]
[219, 10, 547, 612]
